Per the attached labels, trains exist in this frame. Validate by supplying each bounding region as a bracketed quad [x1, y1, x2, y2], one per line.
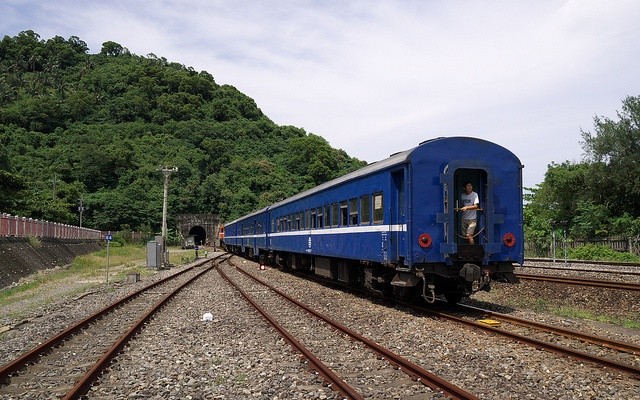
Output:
[219, 137, 524, 303]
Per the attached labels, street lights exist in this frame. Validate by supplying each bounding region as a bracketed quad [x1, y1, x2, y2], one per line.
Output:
[157, 165, 179, 262]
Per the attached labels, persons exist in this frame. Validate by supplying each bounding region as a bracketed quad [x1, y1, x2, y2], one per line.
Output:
[460, 182, 479, 244]
